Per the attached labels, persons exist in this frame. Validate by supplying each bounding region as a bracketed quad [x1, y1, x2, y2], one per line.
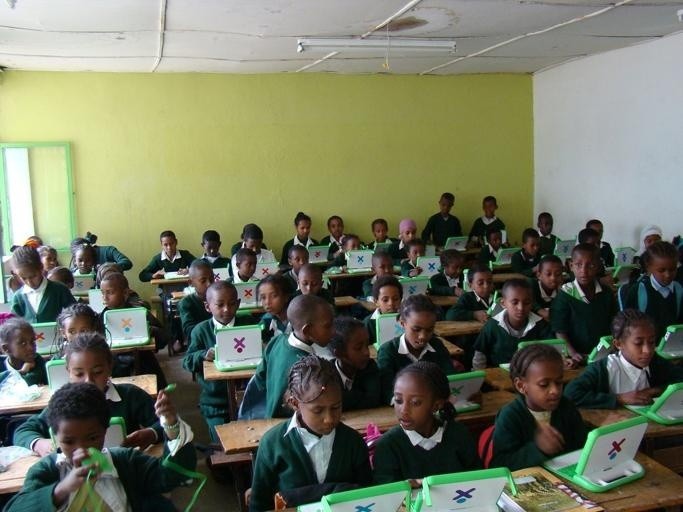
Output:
[4, 382, 197, 511]
[242, 356, 373, 511]
[0, 188, 682, 420]
[492, 342, 590, 466]
[371, 362, 479, 484]
[11, 333, 197, 476]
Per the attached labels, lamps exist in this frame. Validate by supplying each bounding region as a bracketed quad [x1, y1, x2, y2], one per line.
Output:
[296, 32, 458, 55]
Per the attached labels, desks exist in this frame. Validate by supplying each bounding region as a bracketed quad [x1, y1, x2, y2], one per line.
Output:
[585, 412, 683, 477]
[0, 297, 160, 493]
[202, 245, 683, 422]
[281, 445, 683, 512]
[150, 275, 191, 329]
[214, 390, 539, 484]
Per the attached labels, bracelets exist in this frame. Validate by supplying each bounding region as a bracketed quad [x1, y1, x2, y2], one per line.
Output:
[159, 421, 180, 431]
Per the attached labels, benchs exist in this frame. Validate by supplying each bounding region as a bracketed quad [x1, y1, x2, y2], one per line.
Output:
[209, 453, 252, 508]
[149, 295, 174, 352]
